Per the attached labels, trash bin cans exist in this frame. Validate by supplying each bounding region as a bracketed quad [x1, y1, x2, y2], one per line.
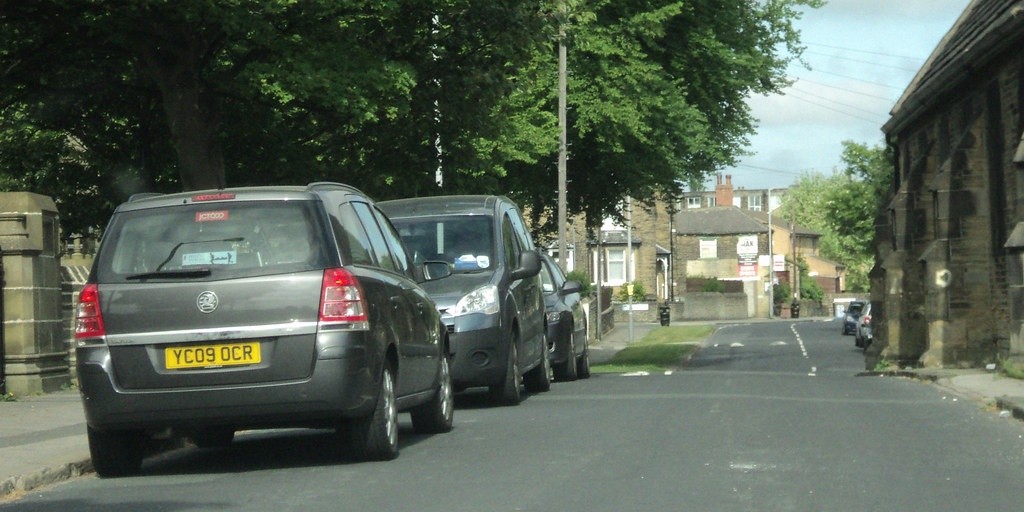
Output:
[791, 303, 799, 319]
[660, 306, 671, 328]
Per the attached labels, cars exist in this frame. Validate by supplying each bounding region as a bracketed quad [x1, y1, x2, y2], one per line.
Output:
[538, 248, 591, 383]
[371, 191, 553, 405]
[855, 301, 873, 351]
[842, 300, 868, 336]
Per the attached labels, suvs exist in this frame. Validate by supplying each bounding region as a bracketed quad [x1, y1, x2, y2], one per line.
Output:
[74, 181, 456, 477]
